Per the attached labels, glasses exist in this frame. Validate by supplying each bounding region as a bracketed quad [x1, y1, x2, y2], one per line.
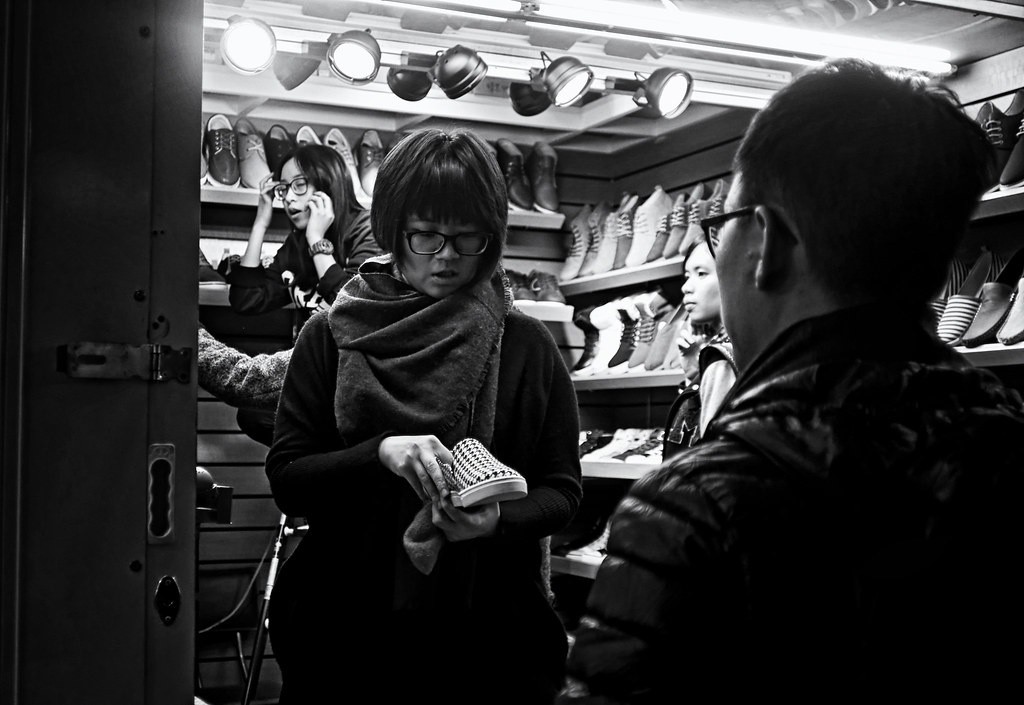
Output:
[401, 229, 494, 256]
[700, 205, 798, 258]
[273, 178, 309, 201]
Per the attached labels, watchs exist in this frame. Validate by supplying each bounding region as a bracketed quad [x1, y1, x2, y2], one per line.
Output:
[308, 239, 334, 256]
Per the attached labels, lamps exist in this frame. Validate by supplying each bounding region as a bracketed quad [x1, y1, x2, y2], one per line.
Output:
[326, 29, 381, 87]
[221, 14, 279, 76]
[634, 67, 694, 120]
[537, 50, 593, 107]
[507, 82, 550, 117]
[387, 67, 431, 101]
[274, 54, 322, 90]
[433, 45, 490, 99]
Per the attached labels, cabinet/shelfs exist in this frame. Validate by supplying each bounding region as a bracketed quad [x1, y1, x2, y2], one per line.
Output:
[200, 186, 1024, 579]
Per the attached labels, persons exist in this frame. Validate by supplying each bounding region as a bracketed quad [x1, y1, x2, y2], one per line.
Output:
[662, 237, 739, 461]
[197, 61, 1023, 705]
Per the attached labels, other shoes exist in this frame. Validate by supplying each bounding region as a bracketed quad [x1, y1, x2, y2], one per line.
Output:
[551, 86, 1024, 566]
[196, 112, 567, 302]
[437, 438, 527, 511]
